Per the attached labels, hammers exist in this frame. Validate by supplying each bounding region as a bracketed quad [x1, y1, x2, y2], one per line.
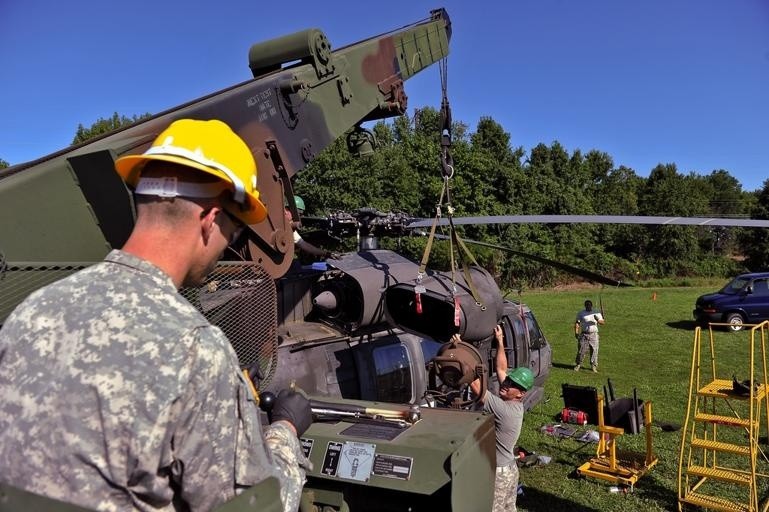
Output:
[308, 399, 421, 424]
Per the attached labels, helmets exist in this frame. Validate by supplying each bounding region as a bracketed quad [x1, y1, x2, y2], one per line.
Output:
[505, 367, 534, 391]
[114, 118, 268, 224]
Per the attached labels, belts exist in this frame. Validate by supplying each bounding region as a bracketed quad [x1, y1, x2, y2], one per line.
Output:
[496, 466, 510, 473]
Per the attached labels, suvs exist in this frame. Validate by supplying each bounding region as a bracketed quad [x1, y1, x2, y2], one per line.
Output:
[693, 272, 769, 332]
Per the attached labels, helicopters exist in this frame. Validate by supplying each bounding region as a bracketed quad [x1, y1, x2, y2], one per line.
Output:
[269, 200, 768, 418]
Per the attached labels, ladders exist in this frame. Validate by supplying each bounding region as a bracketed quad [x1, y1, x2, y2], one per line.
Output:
[677, 319, 769, 512]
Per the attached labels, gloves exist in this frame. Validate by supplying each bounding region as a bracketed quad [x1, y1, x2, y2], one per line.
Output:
[271, 389, 313, 438]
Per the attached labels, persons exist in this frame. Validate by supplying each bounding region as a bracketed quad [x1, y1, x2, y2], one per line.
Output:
[0, 116, 318, 512]
[450, 324, 535, 512]
[572, 299, 606, 374]
[278, 194, 333, 258]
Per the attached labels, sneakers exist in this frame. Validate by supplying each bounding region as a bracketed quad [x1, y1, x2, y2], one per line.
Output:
[592, 367, 597, 373]
[574, 365, 580, 372]
